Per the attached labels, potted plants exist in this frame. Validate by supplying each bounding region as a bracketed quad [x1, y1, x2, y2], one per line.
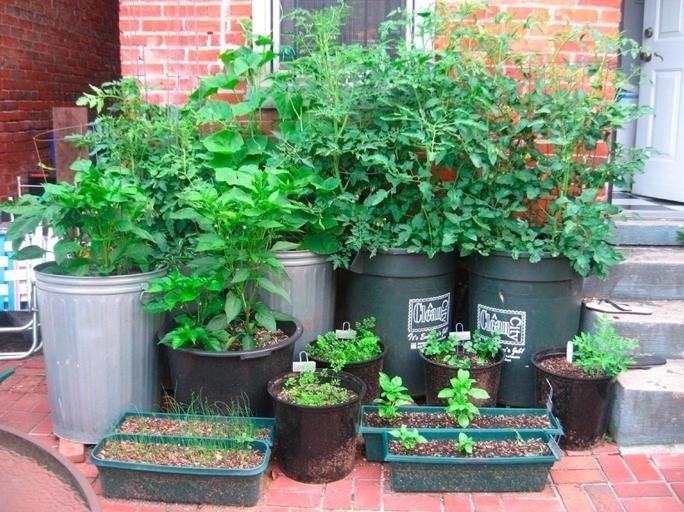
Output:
[0, 0, 663, 503]
